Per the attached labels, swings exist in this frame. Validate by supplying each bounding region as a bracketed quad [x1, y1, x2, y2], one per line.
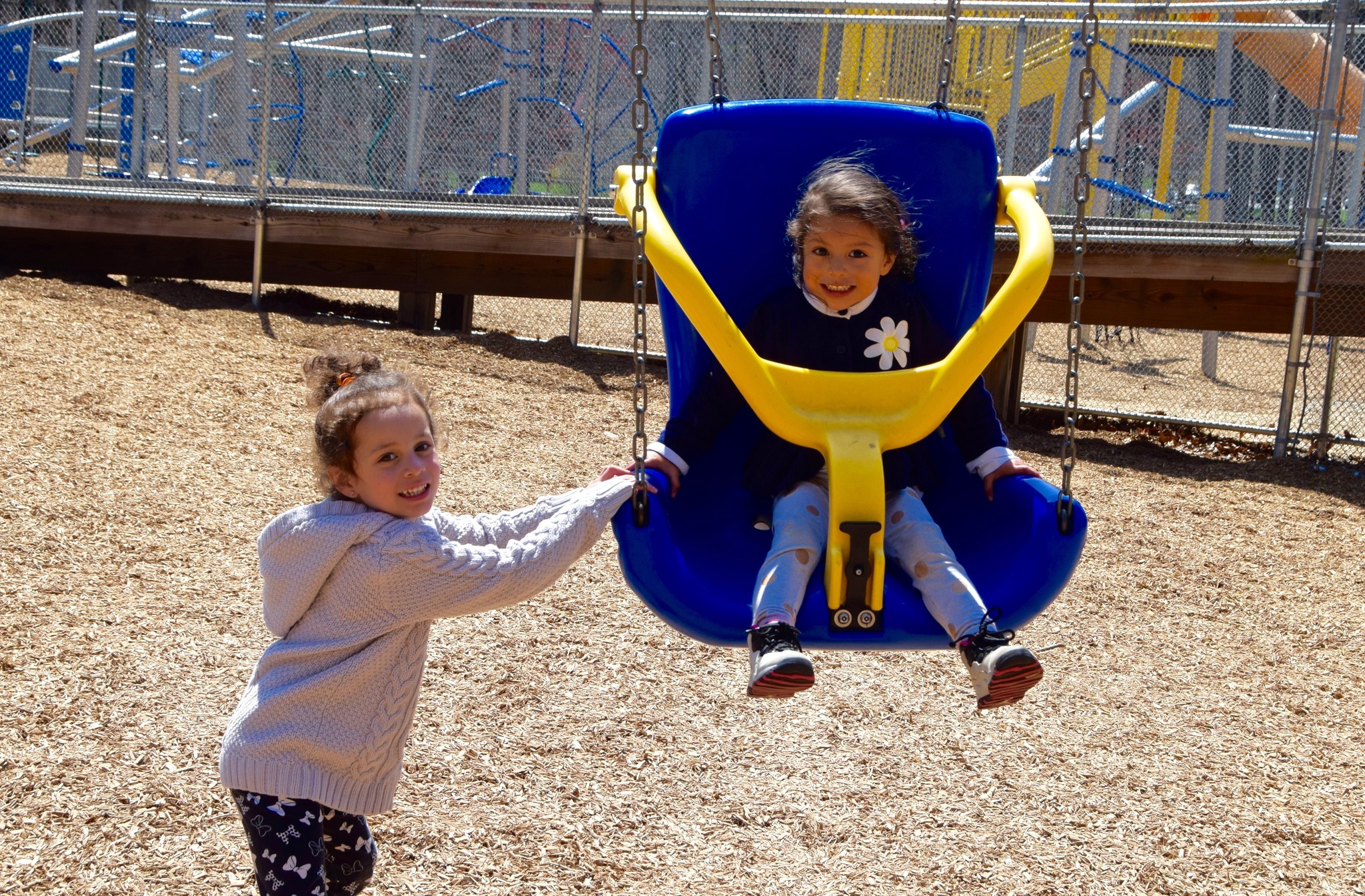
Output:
[609, 2, 1091, 654]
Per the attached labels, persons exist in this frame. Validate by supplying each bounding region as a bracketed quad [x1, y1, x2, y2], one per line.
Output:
[626, 157, 1043, 709]
[219, 354, 658, 896]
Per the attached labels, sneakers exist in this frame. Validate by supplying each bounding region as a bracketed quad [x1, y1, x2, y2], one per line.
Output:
[948, 611, 1043, 709]
[746, 623, 816, 701]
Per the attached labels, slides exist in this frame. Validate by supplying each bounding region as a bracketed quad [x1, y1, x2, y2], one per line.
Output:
[1228, 0, 1365, 167]
